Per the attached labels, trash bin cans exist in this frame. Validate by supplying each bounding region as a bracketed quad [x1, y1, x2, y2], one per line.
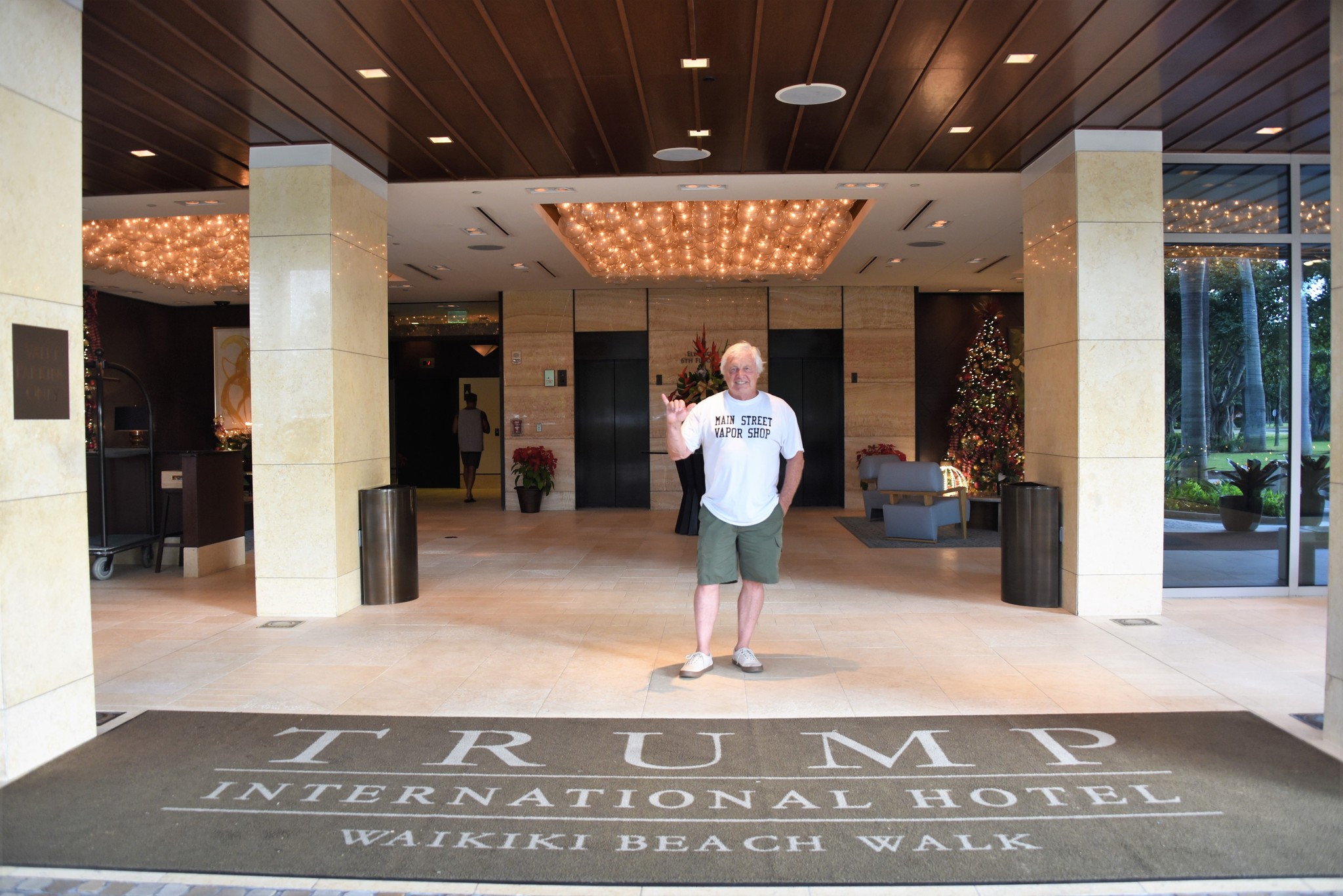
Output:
[998, 481, 1060, 607]
[359, 482, 419, 606]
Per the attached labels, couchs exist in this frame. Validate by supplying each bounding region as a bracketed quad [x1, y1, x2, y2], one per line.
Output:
[859, 454, 902, 522]
[877, 461, 970, 543]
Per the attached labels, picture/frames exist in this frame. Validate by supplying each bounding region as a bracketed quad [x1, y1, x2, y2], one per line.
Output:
[213, 326, 251, 438]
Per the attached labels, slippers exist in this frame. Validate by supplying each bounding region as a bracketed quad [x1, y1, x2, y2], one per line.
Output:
[464, 499, 476, 503]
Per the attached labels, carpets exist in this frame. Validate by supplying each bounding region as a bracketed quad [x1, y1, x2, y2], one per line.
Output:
[833, 516, 1001, 549]
[0, 710, 1343, 886]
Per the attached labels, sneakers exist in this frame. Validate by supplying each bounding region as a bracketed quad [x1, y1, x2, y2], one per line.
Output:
[679, 651, 714, 678]
[732, 646, 763, 672]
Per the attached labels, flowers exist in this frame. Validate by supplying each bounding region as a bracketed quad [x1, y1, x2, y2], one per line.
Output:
[511, 446, 557, 496]
[667, 322, 729, 408]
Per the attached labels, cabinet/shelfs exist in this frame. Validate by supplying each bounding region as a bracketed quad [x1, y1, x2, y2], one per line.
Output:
[84, 347, 162, 581]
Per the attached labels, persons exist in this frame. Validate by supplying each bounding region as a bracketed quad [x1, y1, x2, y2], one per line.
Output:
[452, 392, 490, 502]
[661, 339, 805, 678]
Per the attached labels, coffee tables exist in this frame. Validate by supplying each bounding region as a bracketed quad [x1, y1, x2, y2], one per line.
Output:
[965, 492, 1002, 533]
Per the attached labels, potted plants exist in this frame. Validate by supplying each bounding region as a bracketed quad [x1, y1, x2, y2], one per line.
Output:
[1279, 455, 1329, 527]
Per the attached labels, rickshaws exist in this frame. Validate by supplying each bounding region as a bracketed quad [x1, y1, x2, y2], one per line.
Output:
[84, 349, 164, 578]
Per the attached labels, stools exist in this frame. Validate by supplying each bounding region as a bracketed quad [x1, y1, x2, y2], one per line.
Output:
[155, 488, 183, 573]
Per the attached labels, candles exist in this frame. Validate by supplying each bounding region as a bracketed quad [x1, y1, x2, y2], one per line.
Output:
[1218, 459, 1288, 531]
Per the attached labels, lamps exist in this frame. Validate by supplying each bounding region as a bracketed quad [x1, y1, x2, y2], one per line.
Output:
[554, 199, 857, 284]
[469, 345, 499, 357]
[114, 406, 155, 447]
[82, 212, 250, 296]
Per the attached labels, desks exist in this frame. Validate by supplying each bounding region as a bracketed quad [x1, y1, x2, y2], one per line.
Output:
[1163, 526, 1329, 586]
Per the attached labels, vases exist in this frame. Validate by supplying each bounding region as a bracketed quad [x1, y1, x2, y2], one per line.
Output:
[513, 486, 543, 514]
[675, 449, 707, 536]
